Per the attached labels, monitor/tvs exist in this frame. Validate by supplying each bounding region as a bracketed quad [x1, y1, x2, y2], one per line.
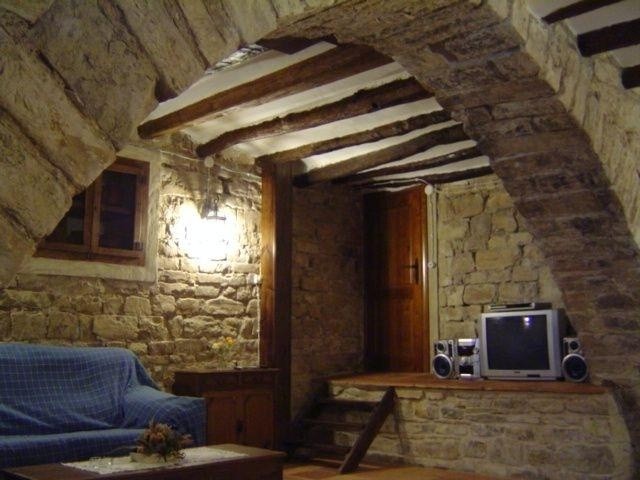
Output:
[479, 309, 563, 380]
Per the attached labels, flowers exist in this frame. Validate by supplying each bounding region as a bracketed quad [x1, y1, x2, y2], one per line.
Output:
[129, 420, 195, 465]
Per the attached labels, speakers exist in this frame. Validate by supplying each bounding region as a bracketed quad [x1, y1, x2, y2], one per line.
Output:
[561, 339, 589, 382]
[432, 340, 480, 379]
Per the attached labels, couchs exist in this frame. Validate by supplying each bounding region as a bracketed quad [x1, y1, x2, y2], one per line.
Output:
[1, 342, 209, 467]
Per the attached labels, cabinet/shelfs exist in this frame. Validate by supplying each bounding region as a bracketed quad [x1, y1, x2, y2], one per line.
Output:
[171, 370, 275, 452]
